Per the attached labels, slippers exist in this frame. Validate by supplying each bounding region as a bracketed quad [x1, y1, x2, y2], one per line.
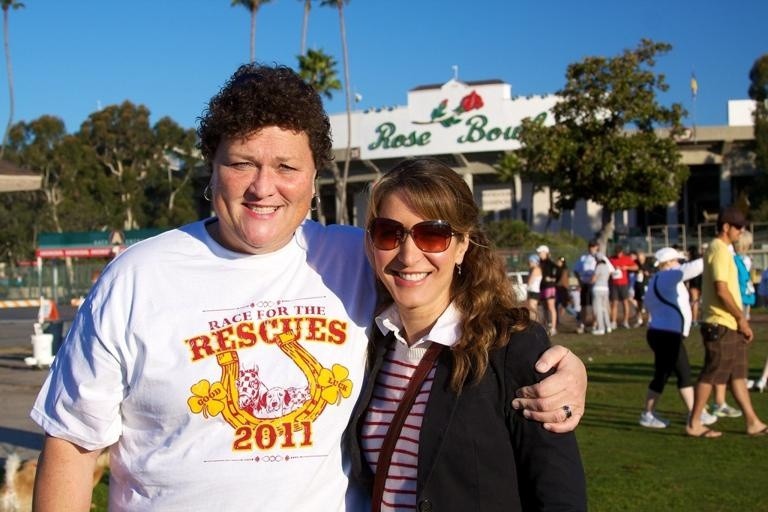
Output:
[684, 429, 726, 439]
[745, 424, 768, 435]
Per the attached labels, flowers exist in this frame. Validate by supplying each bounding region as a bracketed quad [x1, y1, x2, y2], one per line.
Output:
[410, 90, 484, 128]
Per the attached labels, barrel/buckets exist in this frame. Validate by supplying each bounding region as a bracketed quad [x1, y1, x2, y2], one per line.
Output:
[29, 333, 53, 358]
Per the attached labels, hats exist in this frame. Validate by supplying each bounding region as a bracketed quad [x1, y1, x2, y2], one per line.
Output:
[536, 245, 549, 254]
[718, 206, 751, 227]
[648, 245, 689, 268]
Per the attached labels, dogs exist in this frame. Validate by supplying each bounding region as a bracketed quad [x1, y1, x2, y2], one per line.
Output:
[0, 444, 111, 512]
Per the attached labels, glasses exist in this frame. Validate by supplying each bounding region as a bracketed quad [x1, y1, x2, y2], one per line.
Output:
[365, 215, 464, 254]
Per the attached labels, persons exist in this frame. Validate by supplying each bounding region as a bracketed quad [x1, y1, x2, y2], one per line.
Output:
[29, 60, 589, 512]
[526, 241, 653, 336]
[347, 157, 588, 512]
[643, 208, 767, 438]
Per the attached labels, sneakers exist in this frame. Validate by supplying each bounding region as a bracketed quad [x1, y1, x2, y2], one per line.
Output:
[711, 402, 743, 419]
[746, 375, 764, 394]
[638, 412, 671, 428]
[689, 407, 719, 425]
[545, 320, 645, 338]
